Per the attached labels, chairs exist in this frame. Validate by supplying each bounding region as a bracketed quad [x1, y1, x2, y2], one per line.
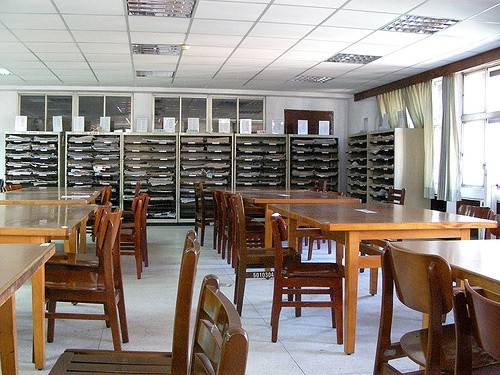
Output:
[0, 174, 500, 375]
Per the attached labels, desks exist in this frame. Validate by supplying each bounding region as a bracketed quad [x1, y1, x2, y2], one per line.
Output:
[0, 240, 58, 375]
[0, 183, 107, 255]
[266, 200, 499, 354]
[238, 190, 363, 279]
[390, 238, 500, 370]
[0, 201, 100, 368]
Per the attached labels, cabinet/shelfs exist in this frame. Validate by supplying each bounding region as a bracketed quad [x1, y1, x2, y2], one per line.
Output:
[63, 130, 123, 232]
[291, 134, 341, 229]
[120, 132, 180, 225]
[234, 132, 290, 223]
[178, 132, 234, 224]
[344, 132, 369, 201]
[3, 131, 64, 188]
[368, 125, 426, 207]
